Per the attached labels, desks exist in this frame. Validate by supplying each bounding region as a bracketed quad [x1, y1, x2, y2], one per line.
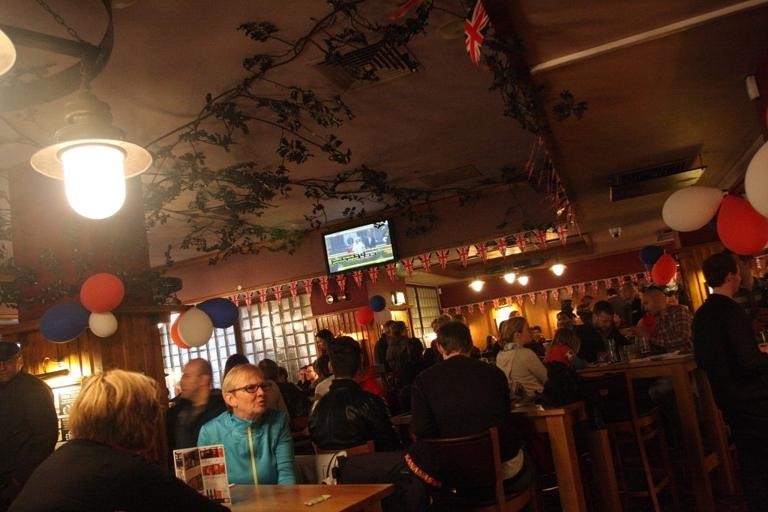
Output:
[229, 484, 397, 511]
[579, 351, 738, 510]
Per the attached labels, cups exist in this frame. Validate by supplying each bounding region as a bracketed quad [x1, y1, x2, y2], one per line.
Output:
[603, 336, 652, 365]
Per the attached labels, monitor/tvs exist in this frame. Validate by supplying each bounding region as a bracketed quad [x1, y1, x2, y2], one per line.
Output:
[321, 218, 395, 274]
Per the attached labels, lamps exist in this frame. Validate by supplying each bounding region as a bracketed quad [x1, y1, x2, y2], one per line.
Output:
[325, 290, 348, 306]
[34, 357, 72, 385]
[0, 0, 158, 224]
[467, 227, 568, 293]
[390, 288, 406, 307]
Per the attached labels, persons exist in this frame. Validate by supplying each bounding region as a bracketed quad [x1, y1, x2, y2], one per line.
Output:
[695, 252, 767, 512]
[0, 342, 59, 512]
[6, 369, 231, 511]
[721, 248, 767, 335]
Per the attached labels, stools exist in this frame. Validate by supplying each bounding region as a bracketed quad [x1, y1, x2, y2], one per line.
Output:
[289, 371, 683, 510]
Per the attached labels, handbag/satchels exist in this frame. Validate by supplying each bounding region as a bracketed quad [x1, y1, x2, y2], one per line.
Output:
[334, 453, 431, 511]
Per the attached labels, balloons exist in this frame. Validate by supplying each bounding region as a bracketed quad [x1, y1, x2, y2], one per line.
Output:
[88, 311, 119, 338]
[640, 245, 663, 264]
[744, 141, 768, 216]
[79, 272, 125, 312]
[39, 302, 89, 343]
[651, 265, 666, 286]
[656, 253, 676, 280]
[717, 193, 768, 256]
[662, 185, 728, 231]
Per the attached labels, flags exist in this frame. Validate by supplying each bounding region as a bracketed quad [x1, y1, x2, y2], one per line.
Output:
[463, 0, 490, 68]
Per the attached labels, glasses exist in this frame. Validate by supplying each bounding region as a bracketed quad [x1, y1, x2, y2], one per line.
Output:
[229, 381, 273, 395]
